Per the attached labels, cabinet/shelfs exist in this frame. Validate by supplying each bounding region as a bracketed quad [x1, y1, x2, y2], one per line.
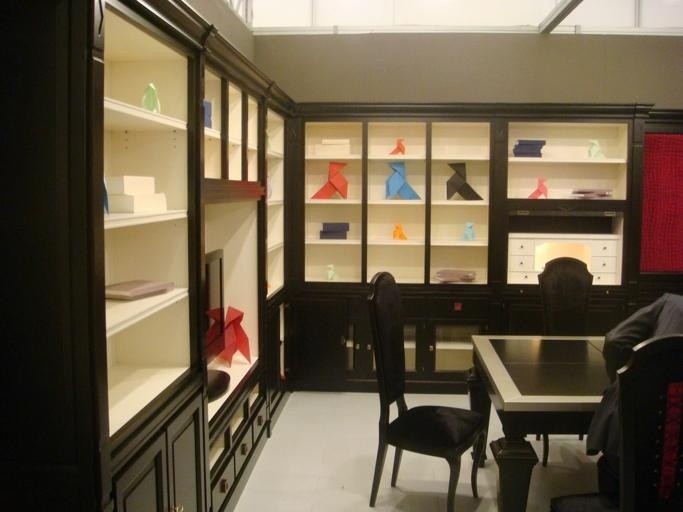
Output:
[297, 102, 655, 394]
[1, 0, 295, 512]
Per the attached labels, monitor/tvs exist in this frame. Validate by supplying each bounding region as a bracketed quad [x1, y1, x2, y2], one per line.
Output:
[205, 248, 230, 401]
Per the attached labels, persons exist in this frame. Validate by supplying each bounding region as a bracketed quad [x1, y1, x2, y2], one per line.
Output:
[580, 289, 683, 512]
